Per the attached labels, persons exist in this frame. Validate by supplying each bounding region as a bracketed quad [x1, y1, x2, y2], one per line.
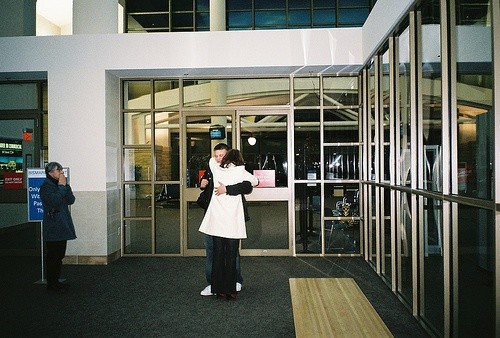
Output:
[198, 148, 259, 301]
[39, 161, 76, 289]
[199, 144, 252, 296]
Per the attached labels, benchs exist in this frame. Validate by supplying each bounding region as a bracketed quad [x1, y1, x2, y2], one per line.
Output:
[288, 277, 396, 338]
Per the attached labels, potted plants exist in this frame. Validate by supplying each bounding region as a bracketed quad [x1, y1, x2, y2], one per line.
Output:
[128, 163, 142, 200]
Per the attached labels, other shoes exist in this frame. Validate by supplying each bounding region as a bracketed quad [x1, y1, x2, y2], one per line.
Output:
[235, 282, 242, 292]
[226, 293, 237, 303]
[216, 292, 225, 300]
[47, 284, 63, 291]
[200, 284, 216, 296]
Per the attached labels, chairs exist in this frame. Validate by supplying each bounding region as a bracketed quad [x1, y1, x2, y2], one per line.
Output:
[146, 184, 180, 209]
[317, 208, 361, 254]
